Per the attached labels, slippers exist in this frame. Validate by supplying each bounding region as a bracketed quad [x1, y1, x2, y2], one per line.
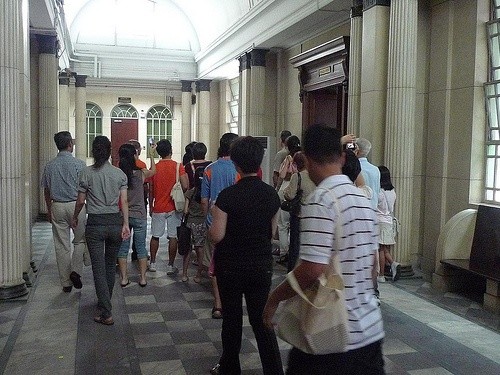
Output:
[120, 279, 132, 287]
[139, 281, 147, 287]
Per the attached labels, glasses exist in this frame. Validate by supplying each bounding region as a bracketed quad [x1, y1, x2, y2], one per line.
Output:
[135, 149, 139, 152]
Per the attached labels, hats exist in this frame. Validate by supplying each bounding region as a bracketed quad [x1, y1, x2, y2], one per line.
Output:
[280, 130, 291, 137]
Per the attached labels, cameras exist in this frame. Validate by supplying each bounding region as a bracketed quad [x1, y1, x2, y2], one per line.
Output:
[149, 138, 155, 147]
[345, 143, 357, 151]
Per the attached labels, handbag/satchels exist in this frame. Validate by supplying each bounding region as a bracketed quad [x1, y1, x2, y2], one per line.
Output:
[394, 217, 400, 242]
[275, 187, 350, 354]
[176, 214, 192, 254]
[281, 172, 303, 213]
[171, 163, 185, 212]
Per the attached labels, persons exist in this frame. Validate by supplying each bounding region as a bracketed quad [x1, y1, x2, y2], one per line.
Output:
[69, 135, 131, 326]
[40, 131, 88, 292]
[117, 123, 402, 375]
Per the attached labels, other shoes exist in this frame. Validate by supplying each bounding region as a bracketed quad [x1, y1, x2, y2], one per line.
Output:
[63, 287, 72, 292]
[149, 263, 157, 272]
[390, 261, 401, 282]
[166, 264, 179, 275]
[182, 277, 189, 282]
[191, 259, 199, 265]
[376, 275, 385, 283]
[210, 364, 224, 375]
[276, 254, 288, 266]
[194, 275, 200, 283]
[93, 316, 114, 325]
[69, 271, 83, 289]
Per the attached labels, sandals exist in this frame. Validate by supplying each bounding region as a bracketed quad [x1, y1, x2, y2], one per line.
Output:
[211, 305, 223, 319]
